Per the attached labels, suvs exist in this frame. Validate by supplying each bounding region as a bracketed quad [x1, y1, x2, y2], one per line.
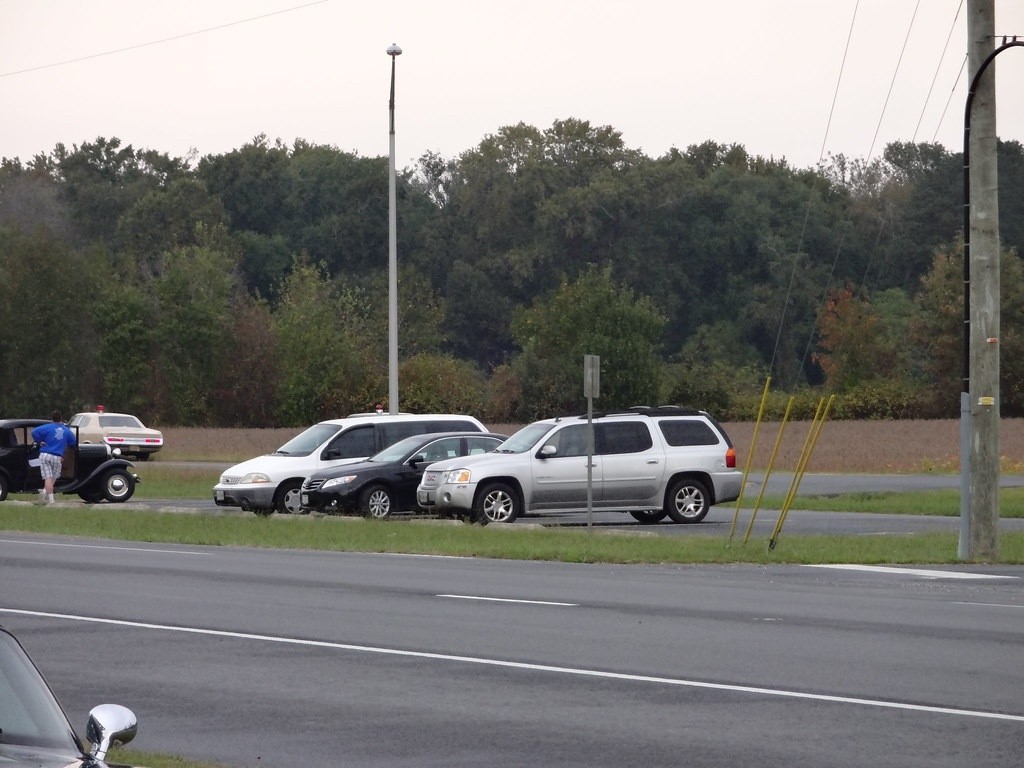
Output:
[416, 405, 744, 529]
[212, 404, 491, 516]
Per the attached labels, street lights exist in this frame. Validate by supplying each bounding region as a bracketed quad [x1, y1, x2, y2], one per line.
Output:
[384, 41, 403, 420]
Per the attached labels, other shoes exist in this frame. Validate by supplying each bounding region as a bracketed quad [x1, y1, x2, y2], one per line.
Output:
[39, 491, 55, 504]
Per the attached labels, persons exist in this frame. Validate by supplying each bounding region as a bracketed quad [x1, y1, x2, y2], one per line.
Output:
[32, 410, 76, 504]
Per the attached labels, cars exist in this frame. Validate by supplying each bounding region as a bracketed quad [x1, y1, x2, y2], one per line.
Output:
[298, 433, 512, 520]
[0, 627, 137, 768]
[0, 419, 142, 504]
[66, 405, 164, 460]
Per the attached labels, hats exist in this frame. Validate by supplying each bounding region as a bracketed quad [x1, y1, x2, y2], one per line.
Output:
[51, 410, 62, 418]
